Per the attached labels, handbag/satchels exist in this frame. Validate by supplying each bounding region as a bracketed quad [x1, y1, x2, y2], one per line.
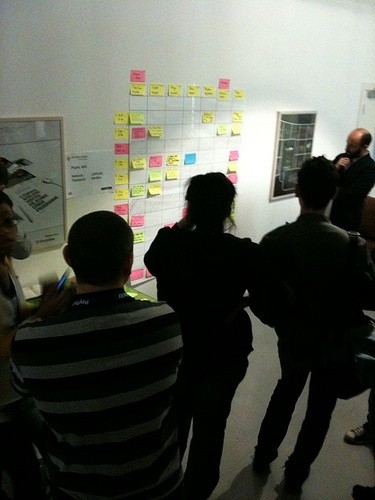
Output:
[318, 313, 375, 400]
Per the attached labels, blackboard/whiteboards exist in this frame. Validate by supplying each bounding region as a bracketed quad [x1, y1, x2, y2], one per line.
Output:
[1, 116, 69, 254]
[270, 112, 317, 202]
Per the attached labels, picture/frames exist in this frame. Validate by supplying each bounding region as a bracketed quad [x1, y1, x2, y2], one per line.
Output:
[0, 116, 68, 256]
[269, 110, 318, 203]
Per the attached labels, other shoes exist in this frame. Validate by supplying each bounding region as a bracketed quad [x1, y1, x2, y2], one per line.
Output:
[343, 422, 375, 445]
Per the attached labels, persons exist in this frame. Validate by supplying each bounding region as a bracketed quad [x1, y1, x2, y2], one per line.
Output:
[143, 172, 261, 499]
[333, 128, 375, 214]
[0, 192, 58, 500]
[252, 156, 368, 499]
[11, 211, 185, 500]
